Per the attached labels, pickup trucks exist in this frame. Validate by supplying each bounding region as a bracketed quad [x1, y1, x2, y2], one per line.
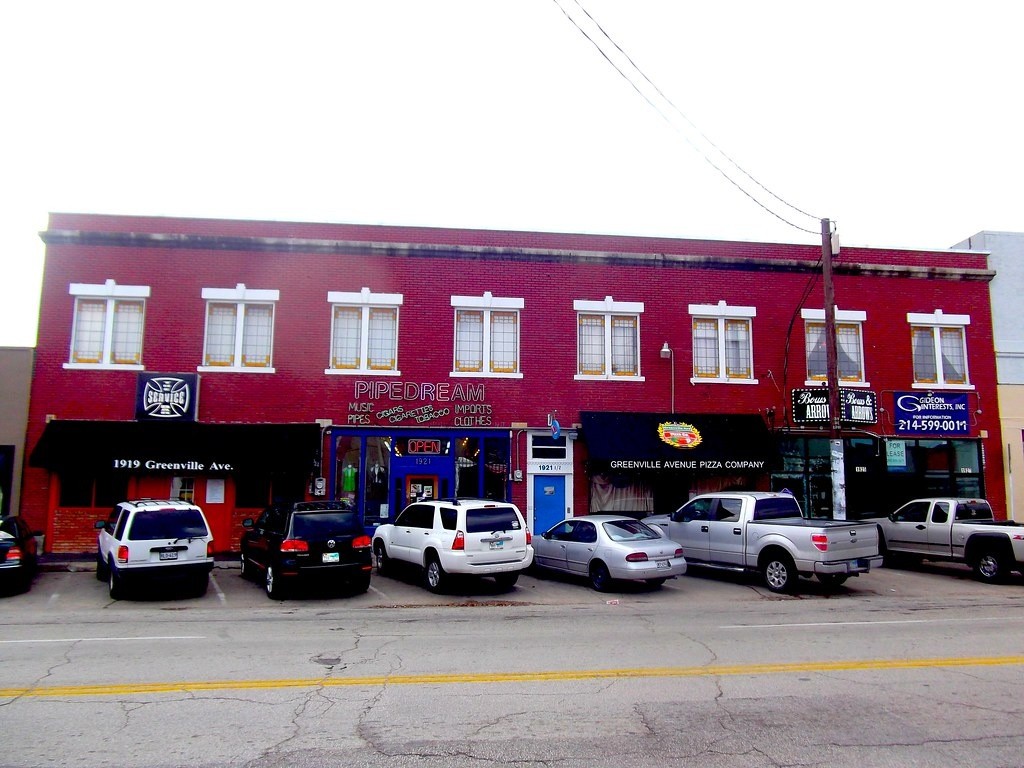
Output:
[640, 490, 884, 594]
[861, 496, 1024, 584]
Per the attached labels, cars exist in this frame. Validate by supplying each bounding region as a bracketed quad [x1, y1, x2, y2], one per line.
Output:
[0, 515, 40, 594]
[529, 515, 687, 593]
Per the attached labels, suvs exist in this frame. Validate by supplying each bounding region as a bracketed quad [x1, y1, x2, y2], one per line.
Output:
[238, 498, 372, 599]
[95, 498, 215, 599]
[371, 495, 535, 593]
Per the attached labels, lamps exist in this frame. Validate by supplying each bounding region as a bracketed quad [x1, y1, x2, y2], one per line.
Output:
[568, 431, 578, 439]
[661, 342, 671, 358]
